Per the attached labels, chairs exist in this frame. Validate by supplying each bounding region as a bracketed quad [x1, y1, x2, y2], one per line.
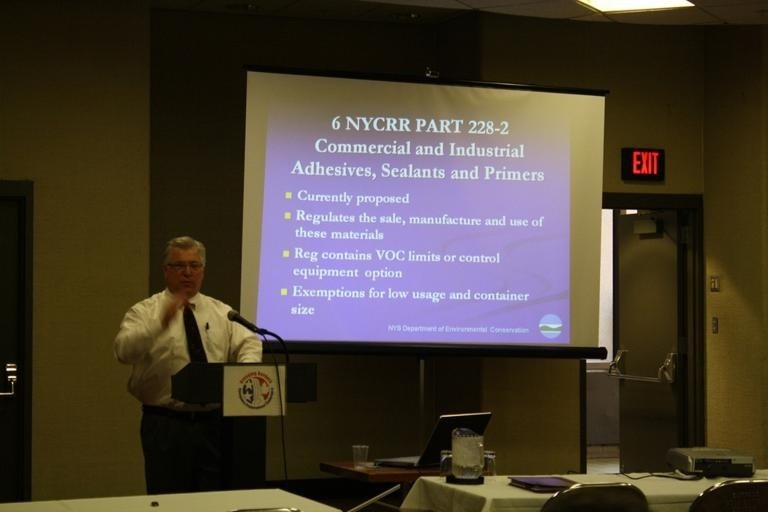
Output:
[688, 478, 768, 511]
[539, 482, 648, 511]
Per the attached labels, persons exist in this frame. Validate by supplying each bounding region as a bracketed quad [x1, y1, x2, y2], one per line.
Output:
[112, 236, 264, 494]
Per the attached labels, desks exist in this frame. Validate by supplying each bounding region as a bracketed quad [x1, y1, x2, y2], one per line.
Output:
[321, 462, 449, 484]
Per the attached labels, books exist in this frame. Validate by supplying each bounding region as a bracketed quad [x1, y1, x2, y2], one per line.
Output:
[506, 474, 580, 493]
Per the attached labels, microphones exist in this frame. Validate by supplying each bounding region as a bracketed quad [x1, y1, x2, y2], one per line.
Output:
[228, 311, 258, 332]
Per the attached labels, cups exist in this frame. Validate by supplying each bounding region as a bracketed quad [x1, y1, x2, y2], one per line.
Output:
[451, 432, 487, 480]
[352, 444, 369, 466]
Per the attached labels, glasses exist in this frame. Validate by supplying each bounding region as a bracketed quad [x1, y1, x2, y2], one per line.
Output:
[167, 261, 203, 272]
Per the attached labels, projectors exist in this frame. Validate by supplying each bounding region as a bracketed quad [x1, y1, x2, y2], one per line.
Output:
[667, 447, 755, 477]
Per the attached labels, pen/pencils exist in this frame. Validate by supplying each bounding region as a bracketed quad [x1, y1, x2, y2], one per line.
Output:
[206, 322, 209, 329]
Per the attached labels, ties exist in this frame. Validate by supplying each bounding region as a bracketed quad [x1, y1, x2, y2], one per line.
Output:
[183, 304, 207, 364]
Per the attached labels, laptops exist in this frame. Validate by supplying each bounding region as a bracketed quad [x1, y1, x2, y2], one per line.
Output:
[375, 411, 492, 467]
